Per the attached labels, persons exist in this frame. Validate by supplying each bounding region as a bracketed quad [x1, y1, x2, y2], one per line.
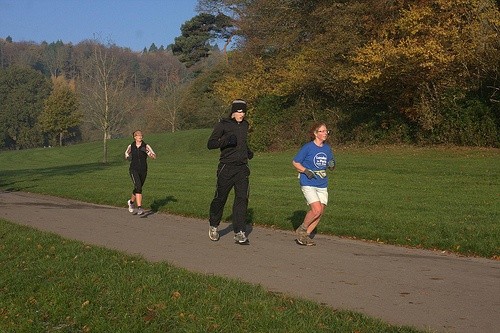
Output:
[292, 121, 336, 245]
[124, 131, 157, 215]
[207, 98, 254, 244]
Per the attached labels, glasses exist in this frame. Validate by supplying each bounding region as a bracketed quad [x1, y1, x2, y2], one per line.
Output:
[317, 130, 330, 134]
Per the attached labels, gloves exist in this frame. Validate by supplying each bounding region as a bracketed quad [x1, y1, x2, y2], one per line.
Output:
[304, 169, 317, 180]
[222, 135, 237, 148]
[248, 148, 254, 160]
[328, 160, 335, 171]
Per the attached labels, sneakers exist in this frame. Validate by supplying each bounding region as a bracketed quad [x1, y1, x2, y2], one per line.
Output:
[233, 231, 249, 244]
[208, 223, 219, 240]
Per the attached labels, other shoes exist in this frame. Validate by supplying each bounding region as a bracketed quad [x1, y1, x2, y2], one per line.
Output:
[138, 209, 144, 215]
[128, 200, 135, 212]
[296, 225, 307, 244]
[306, 233, 316, 245]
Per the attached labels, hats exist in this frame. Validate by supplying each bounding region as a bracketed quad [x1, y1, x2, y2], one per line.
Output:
[231, 99, 247, 115]
